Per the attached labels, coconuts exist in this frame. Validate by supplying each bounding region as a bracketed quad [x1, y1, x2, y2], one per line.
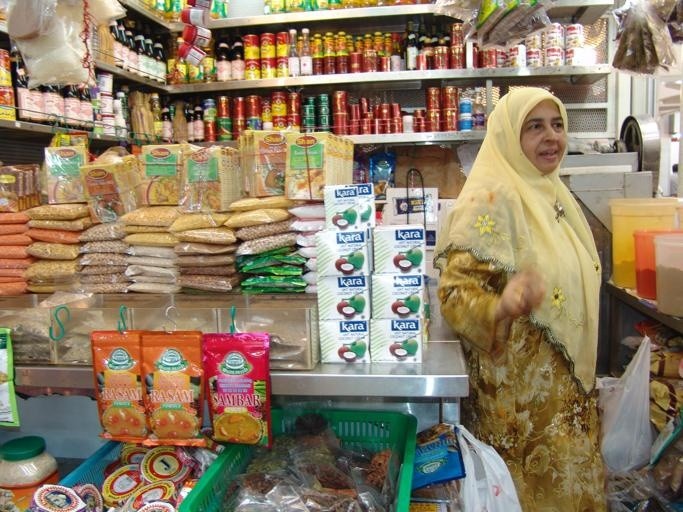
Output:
[332, 204, 422, 362]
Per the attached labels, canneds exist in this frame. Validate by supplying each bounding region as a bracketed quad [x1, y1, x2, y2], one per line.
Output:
[350, 23, 584, 73]
[243, 31, 290, 80]
[202, 85, 459, 142]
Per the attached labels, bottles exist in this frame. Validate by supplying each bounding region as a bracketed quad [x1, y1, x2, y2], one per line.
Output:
[314, 30, 392, 57]
[286, 26, 313, 78]
[392, 32, 451, 70]
[151, 92, 205, 145]
[176, 0, 214, 69]
[0, 434, 61, 512]
[216, 40, 245, 81]
[9, 39, 94, 131]
[110, 16, 167, 86]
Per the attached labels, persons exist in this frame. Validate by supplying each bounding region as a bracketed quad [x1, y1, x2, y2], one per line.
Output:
[429, 85, 609, 510]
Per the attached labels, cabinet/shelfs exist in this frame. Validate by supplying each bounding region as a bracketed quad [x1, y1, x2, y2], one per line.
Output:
[0, 0, 683, 512]
